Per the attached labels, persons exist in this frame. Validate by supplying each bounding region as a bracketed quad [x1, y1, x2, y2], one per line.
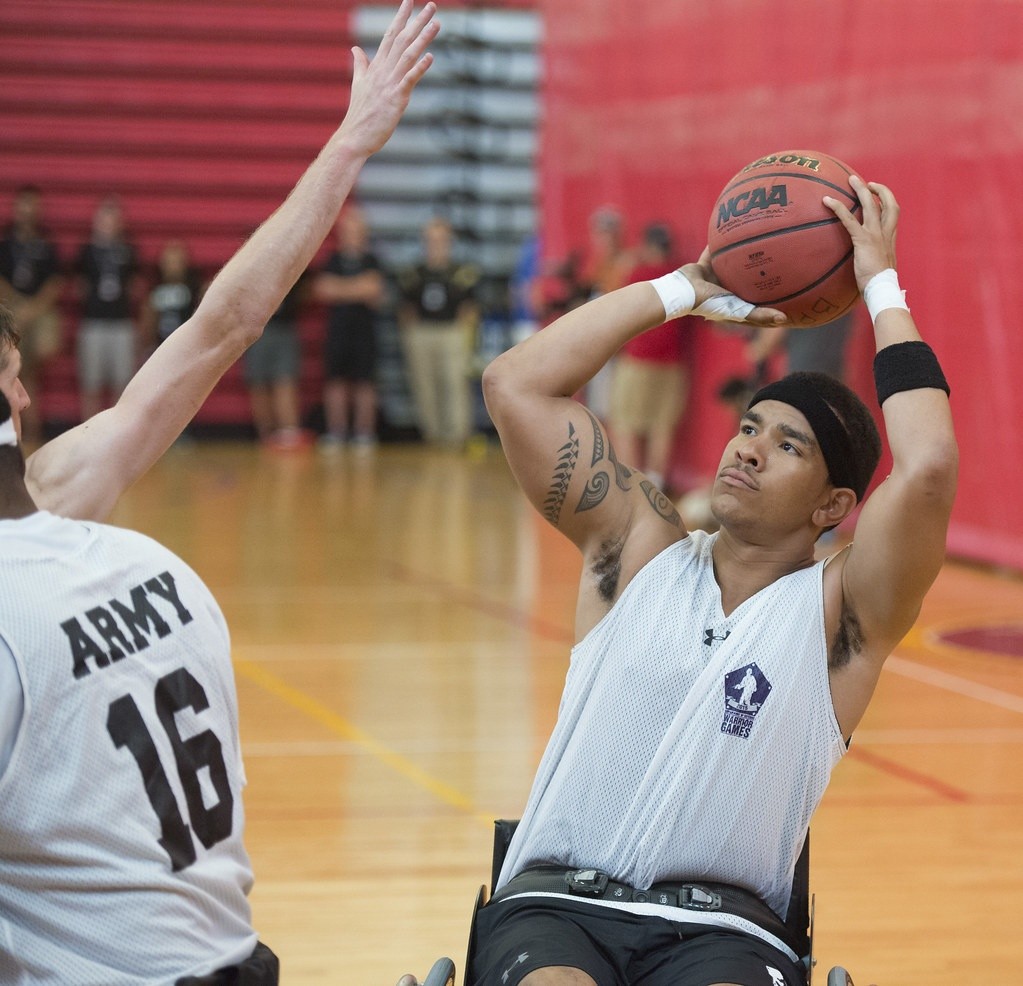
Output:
[0, 0, 439, 523]
[474, 174, 958, 986]
[0, 392, 281, 986]
[0, 183, 857, 493]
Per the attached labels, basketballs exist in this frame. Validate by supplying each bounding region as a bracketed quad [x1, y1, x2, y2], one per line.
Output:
[707, 150, 883, 329]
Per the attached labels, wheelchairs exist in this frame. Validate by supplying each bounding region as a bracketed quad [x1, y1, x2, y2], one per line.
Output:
[394, 815, 856, 986]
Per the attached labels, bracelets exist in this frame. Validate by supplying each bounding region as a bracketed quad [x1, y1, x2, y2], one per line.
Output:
[872, 340, 951, 409]
